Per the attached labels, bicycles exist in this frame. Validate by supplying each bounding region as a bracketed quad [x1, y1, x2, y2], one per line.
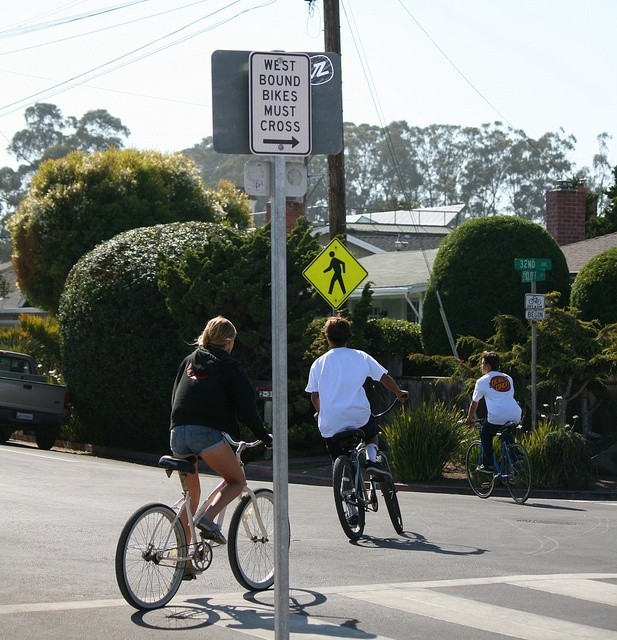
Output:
[115, 432, 290, 608]
[463, 420, 531, 504]
[313, 394, 407, 539]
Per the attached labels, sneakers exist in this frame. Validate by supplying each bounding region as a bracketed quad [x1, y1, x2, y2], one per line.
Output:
[344, 511, 358, 528]
[477, 464, 495, 473]
[197, 516, 227, 544]
[364, 460, 392, 481]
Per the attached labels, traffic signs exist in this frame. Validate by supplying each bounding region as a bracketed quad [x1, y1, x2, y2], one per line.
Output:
[248, 50, 312, 157]
[515, 258, 551, 283]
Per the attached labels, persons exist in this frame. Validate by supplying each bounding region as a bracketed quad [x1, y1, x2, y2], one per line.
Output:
[305, 315, 409, 527]
[169, 315, 273, 578]
[463, 352, 523, 483]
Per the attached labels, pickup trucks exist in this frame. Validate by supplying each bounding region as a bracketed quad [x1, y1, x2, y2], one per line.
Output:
[0, 350, 71, 450]
